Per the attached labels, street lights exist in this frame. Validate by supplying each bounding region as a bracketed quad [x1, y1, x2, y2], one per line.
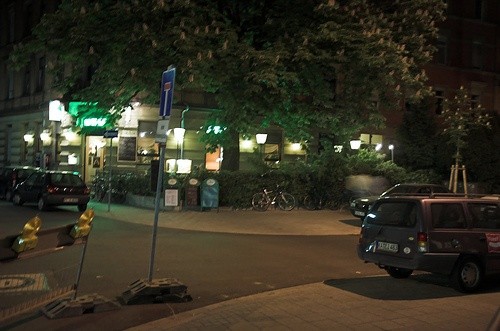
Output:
[389, 145, 394, 164]
[166, 128, 192, 174]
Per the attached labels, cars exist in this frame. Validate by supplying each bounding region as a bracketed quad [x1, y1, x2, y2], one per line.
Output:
[0, 166, 40, 202]
[12, 170, 90, 213]
[347, 183, 455, 221]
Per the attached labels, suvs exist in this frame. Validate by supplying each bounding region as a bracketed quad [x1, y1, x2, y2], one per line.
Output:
[357, 196, 500, 293]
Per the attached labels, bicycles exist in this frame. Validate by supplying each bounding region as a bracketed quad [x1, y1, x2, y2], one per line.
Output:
[92, 177, 127, 204]
[304, 190, 328, 210]
[252, 188, 295, 211]
